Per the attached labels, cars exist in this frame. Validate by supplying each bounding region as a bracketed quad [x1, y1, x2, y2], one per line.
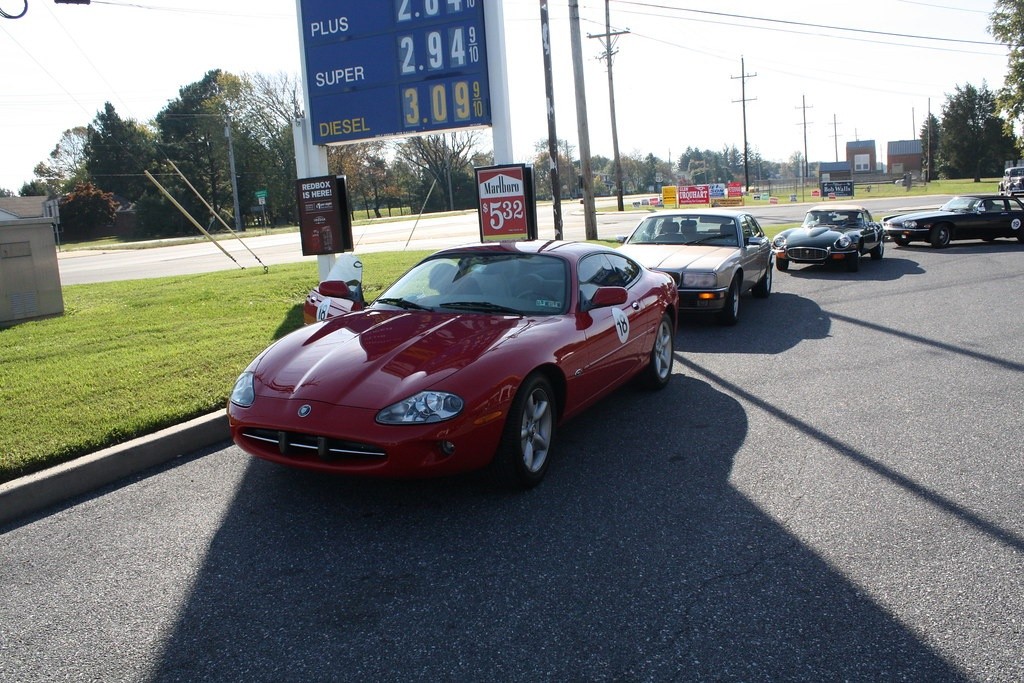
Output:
[997, 166, 1024, 197]
[881, 194, 1024, 249]
[615, 211, 772, 321]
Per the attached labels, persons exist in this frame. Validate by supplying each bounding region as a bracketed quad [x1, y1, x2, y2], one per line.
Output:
[842, 214, 862, 228]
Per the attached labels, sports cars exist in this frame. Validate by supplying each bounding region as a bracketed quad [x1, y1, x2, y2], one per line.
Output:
[227, 238, 680, 492]
[772, 205, 888, 271]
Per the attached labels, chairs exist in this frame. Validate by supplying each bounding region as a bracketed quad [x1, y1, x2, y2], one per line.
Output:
[984, 200, 995, 211]
[471, 274, 511, 297]
[515, 274, 567, 303]
[720, 224, 736, 236]
[655, 222, 683, 242]
[681, 225, 697, 234]
